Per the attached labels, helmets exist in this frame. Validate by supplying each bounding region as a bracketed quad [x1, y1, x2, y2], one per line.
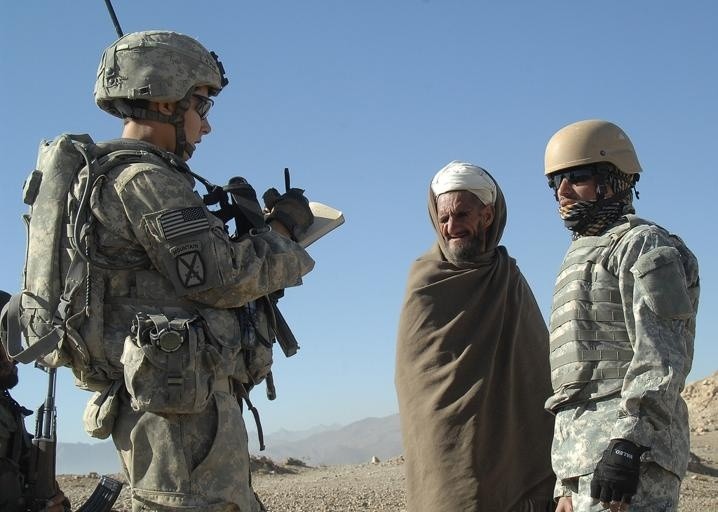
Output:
[540, 115, 645, 182]
[1, 290, 13, 311]
[90, 28, 230, 122]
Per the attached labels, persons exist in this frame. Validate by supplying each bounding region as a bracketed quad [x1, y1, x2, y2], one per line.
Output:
[395, 160, 557, 512]
[77, 30, 314, 512]
[543, 120, 700, 512]
[0, 289, 71, 512]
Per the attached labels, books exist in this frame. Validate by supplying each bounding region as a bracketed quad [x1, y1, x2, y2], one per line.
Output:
[298, 201, 345, 249]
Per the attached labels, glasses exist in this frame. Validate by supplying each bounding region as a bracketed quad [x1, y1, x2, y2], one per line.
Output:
[187, 94, 216, 121]
[551, 169, 594, 191]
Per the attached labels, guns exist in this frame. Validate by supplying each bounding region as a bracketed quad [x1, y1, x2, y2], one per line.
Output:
[229, 178, 301, 399]
[27, 367, 122, 511]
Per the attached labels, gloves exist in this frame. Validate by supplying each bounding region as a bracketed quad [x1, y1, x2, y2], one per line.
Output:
[264, 186, 315, 243]
[588, 436, 652, 504]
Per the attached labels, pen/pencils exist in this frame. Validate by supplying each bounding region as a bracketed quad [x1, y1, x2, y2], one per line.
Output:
[284, 168, 290, 192]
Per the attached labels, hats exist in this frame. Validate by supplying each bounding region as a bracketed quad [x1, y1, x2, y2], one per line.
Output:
[428, 158, 499, 205]
